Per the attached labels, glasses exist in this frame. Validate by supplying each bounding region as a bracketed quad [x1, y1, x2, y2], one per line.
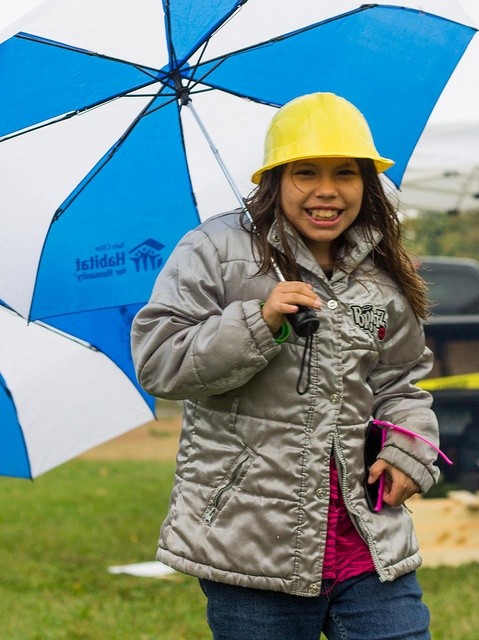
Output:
[363, 420, 453, 513]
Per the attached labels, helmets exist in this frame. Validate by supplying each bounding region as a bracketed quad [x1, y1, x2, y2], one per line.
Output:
[251, 92, 395, 184]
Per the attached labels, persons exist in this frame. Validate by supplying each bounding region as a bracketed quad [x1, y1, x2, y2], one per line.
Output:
[130, 92, 440, 640]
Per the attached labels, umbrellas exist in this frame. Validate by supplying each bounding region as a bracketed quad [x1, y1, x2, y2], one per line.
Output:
[0, 2, 479, 482]
